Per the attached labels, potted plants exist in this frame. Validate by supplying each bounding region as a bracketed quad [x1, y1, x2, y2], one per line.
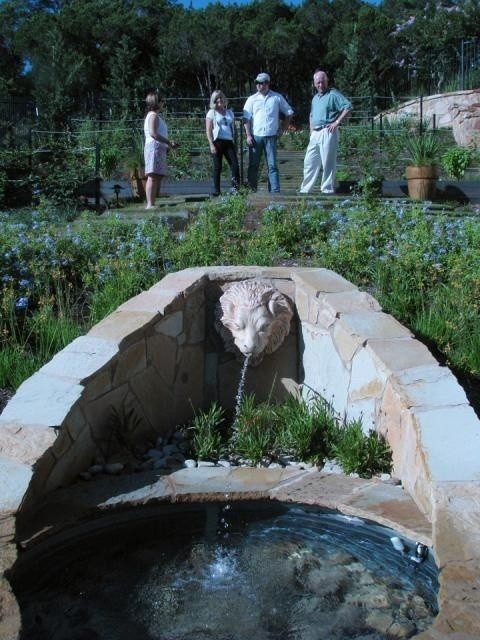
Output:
[336, 170, 359, 193]
[128, 128, 160, 197]
[72, 119, 99, 198]
[400, 130, 442, 201]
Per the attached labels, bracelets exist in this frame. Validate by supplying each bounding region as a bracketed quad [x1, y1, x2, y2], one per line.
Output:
[335, 119, 339, 124]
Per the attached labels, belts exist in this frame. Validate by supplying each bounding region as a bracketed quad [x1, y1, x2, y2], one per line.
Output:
[312, 125, 329, 131]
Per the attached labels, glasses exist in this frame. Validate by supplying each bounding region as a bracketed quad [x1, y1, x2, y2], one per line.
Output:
[254, 82, 264, 85]
[223, 117, 228, 127]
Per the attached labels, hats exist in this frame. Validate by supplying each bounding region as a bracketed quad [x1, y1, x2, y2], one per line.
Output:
[254, 72, 271, 84]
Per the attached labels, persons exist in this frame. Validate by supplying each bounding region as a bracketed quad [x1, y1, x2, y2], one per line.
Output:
[242, 73, 294, 193]
[205, 90, 240, 196]
[143, 91, 177, 209]
[299, 71, 354, 194]
